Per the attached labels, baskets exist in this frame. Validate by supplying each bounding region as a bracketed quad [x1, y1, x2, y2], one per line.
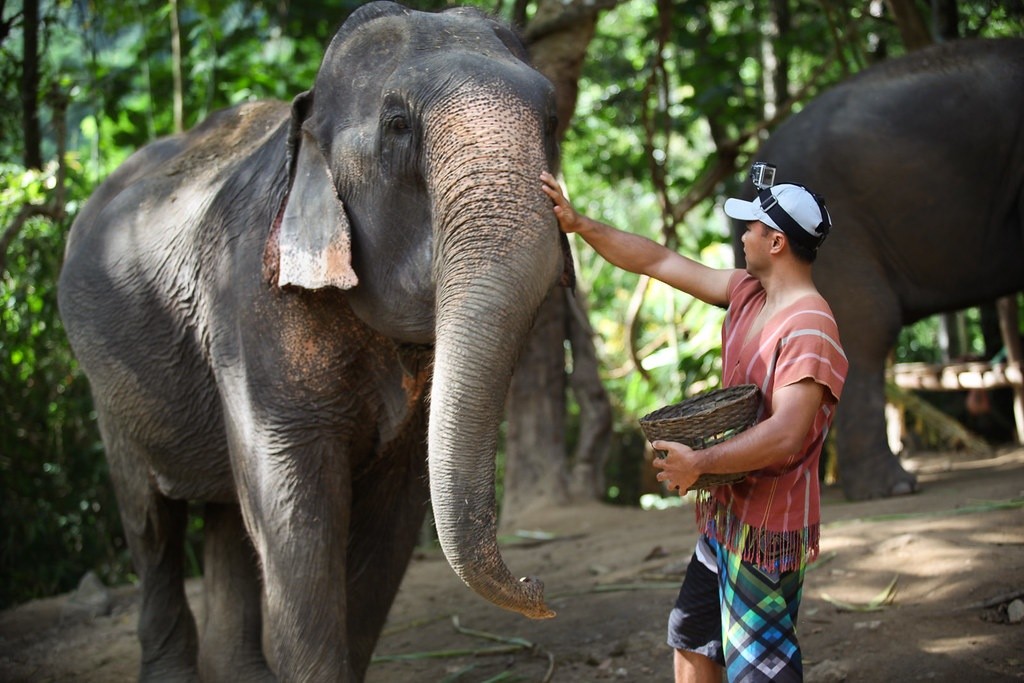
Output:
[638, 382, 766, 491]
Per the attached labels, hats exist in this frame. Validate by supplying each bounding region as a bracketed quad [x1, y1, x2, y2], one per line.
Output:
[725, 185, 831, 238]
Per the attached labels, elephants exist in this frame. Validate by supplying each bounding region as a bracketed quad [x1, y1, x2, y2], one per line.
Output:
[55, 0, 612, 683]
[729, 32, 1024, 500]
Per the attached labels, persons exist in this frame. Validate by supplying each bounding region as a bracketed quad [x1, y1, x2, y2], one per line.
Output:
[539, 171, 848, 683]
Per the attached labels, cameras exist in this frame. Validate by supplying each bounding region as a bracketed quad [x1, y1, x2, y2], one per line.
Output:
[749, 160, 777, 191]
